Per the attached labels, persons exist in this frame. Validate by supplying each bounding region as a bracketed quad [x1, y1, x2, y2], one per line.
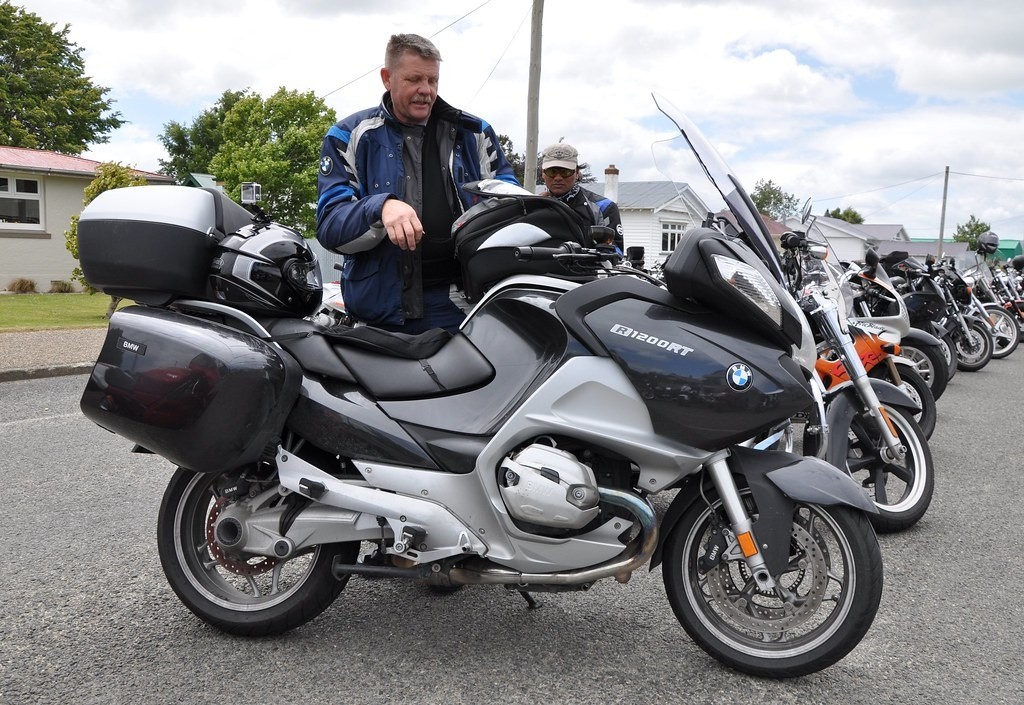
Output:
[535, 143, 624, 260]
[317, 32, 521, 593]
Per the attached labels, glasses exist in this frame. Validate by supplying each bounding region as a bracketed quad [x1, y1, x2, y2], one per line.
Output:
[544, 169, 575, 178]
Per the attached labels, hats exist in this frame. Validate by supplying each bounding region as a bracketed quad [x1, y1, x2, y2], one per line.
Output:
[542, 144, 578, 170]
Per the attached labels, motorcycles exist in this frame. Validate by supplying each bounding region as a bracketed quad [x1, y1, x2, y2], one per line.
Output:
[72, 91, 888, 680]
[768, 198, 1024, 537]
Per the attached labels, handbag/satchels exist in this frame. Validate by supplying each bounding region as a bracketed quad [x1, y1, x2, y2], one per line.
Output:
[452, 195, 597, 301]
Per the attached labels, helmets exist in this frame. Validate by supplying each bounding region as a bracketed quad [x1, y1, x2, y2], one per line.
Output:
[206, 219, 323, 320]
[1012, 255, 1024, 270]
[978, 231, 999, 254]
